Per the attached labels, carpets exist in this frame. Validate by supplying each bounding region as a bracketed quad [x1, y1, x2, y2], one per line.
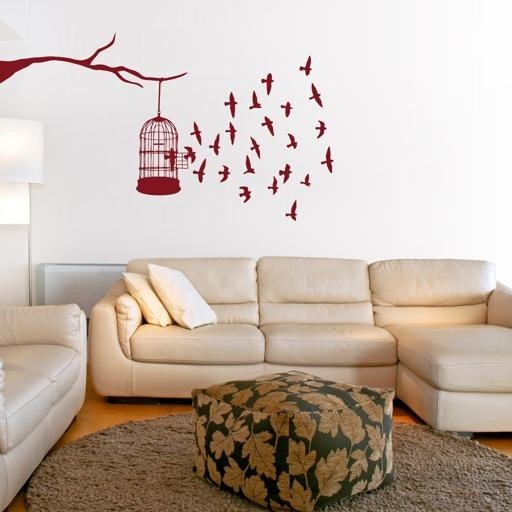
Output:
[24, 412, 511, 511]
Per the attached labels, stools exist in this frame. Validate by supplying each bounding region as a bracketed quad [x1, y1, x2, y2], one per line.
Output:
[188, 370, 395, 511]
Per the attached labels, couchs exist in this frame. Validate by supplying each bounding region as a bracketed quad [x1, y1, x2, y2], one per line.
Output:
[88, 256, 512, 439]
[2, 301, 85, 512]
